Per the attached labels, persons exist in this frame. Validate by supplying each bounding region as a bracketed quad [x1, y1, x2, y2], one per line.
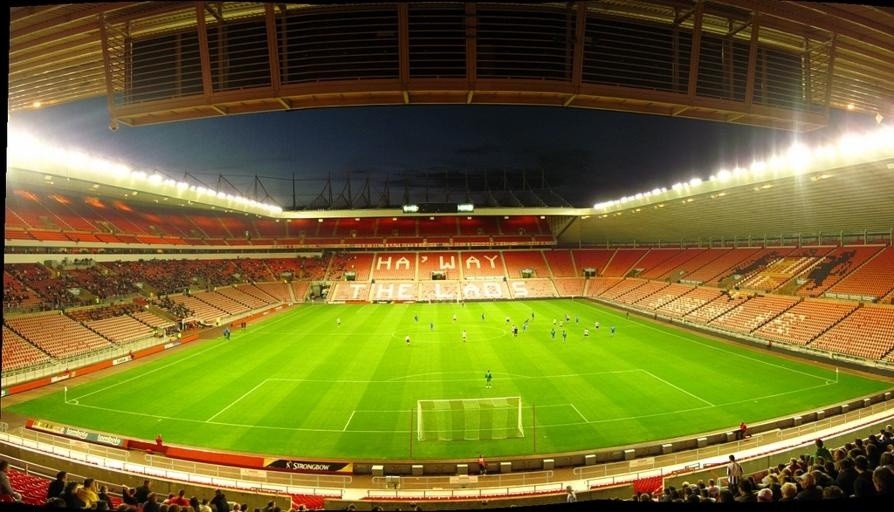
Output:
[484, 370, 493, 389]
[566, 422, 893, 510]
[3, 256, 352, 340]
[405, 300, 616, 345]
[478, 454, 488, 476]
[0, 460, 424, 511]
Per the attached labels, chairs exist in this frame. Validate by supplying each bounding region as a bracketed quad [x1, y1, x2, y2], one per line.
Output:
[0, 454, 694, 512]
